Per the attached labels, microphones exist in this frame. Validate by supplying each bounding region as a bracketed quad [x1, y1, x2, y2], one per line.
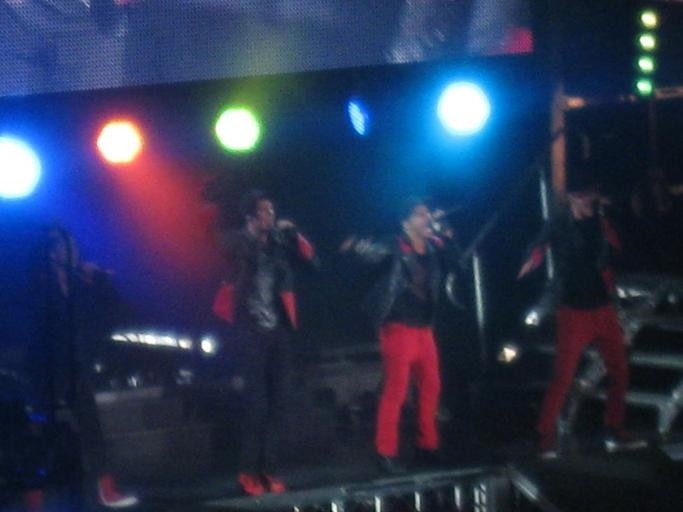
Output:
[274, 215, 296, 246]
[589, 193, 611, 220]
[428, 202, 464, 230]
[72, 262, 115, 280]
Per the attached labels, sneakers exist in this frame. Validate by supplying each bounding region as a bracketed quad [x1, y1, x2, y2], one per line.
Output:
[99, 477, 137, 506]
[266, 476, 283, 493]
[240, 473, 264, 495]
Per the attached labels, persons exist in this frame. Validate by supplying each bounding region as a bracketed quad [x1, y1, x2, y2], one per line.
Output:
[205, 189, 312, 497]
[18, 226, 135, 512]
[340, 196, 453, 474]
[518, 178, 636, 451]
